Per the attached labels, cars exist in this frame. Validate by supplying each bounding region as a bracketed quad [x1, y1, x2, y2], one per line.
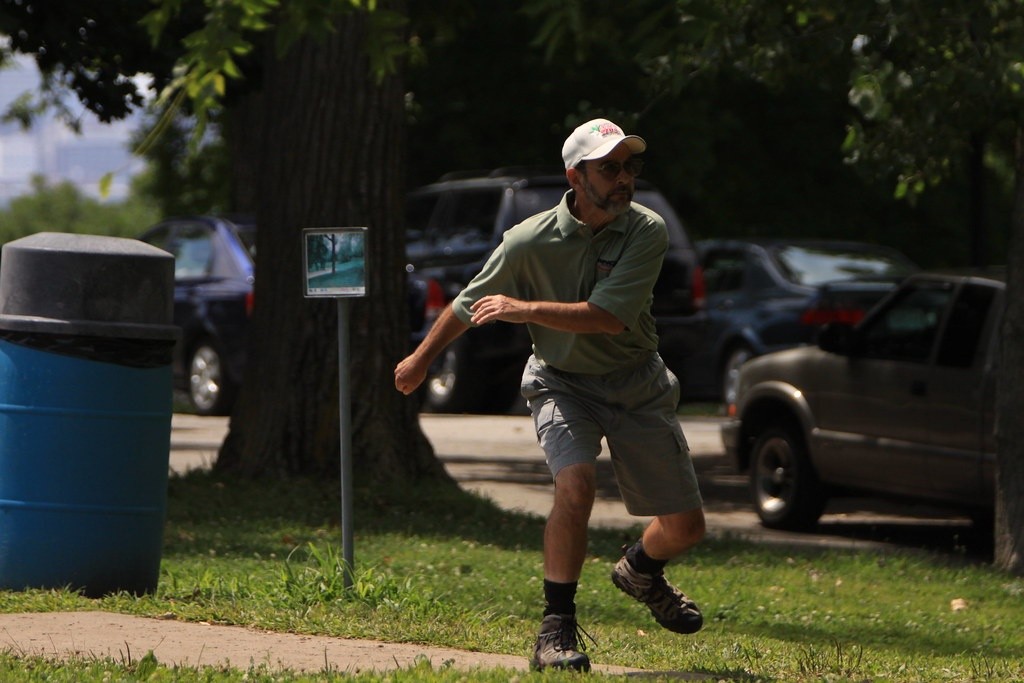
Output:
[133, 212, 451, 418]
[721, 258, 1016, 528]
[687, 235, 923, 414]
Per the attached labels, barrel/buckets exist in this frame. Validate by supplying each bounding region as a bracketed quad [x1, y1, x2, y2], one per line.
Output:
[0, 233, 181, 596]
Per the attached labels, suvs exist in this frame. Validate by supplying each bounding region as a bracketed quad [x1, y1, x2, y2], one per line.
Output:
[400, 169, 712, 413]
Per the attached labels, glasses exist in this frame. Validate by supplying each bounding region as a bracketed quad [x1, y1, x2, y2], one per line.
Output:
[584, 157, 645, 178]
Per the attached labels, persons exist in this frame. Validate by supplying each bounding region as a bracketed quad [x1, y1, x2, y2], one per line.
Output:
[393, 118, 705, 670]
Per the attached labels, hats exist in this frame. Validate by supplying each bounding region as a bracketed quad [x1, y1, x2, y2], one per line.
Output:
[561, 118, 647, 170]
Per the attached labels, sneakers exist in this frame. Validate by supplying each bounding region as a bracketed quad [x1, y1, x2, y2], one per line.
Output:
[611, 554, 704, 635]
[533, 609, 598, 674]
